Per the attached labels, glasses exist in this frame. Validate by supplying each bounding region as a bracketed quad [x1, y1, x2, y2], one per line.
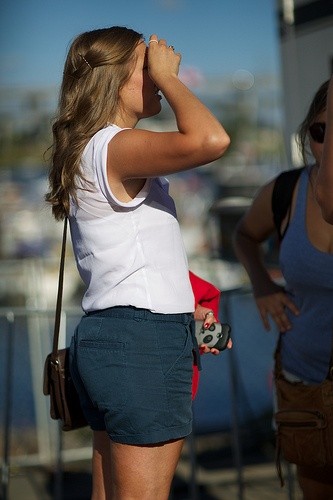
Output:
[306, 121, 326, 144]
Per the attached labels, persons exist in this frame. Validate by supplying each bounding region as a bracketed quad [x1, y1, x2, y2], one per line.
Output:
[232, 79, 333, 500]
[43, 25, 231, 500]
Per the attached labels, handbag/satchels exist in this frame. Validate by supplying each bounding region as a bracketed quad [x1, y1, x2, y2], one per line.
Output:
[42, 346, 87, 432]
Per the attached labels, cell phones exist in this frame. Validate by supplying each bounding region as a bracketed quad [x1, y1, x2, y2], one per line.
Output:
[195, 321, 231, 351]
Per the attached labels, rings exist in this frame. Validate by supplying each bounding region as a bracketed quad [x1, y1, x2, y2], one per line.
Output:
[148, 40, 158, 44]
[206, 310, 214, 316]
[170, 45, 174, 50]
